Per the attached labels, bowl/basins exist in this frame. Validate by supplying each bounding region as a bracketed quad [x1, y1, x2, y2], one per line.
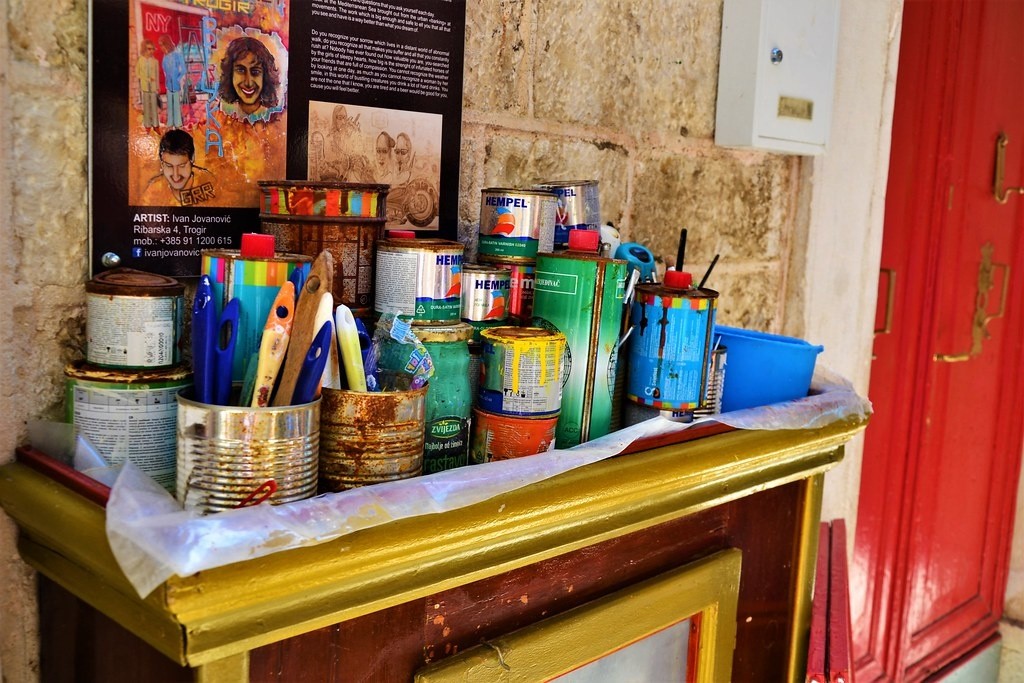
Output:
[714, 326, 824, 413]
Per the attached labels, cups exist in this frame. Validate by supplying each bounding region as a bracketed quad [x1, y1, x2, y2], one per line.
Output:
[320, 373, 427, 496]
[177, 388, 321, 514]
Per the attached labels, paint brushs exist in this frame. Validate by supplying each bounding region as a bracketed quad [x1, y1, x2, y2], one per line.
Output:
[620, 256, 676, 337]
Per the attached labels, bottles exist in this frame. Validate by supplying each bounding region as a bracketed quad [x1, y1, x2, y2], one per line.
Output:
[537, 227, 628, 452]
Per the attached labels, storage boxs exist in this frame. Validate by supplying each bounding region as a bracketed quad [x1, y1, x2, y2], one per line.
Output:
[715, 324, 825, 412]
